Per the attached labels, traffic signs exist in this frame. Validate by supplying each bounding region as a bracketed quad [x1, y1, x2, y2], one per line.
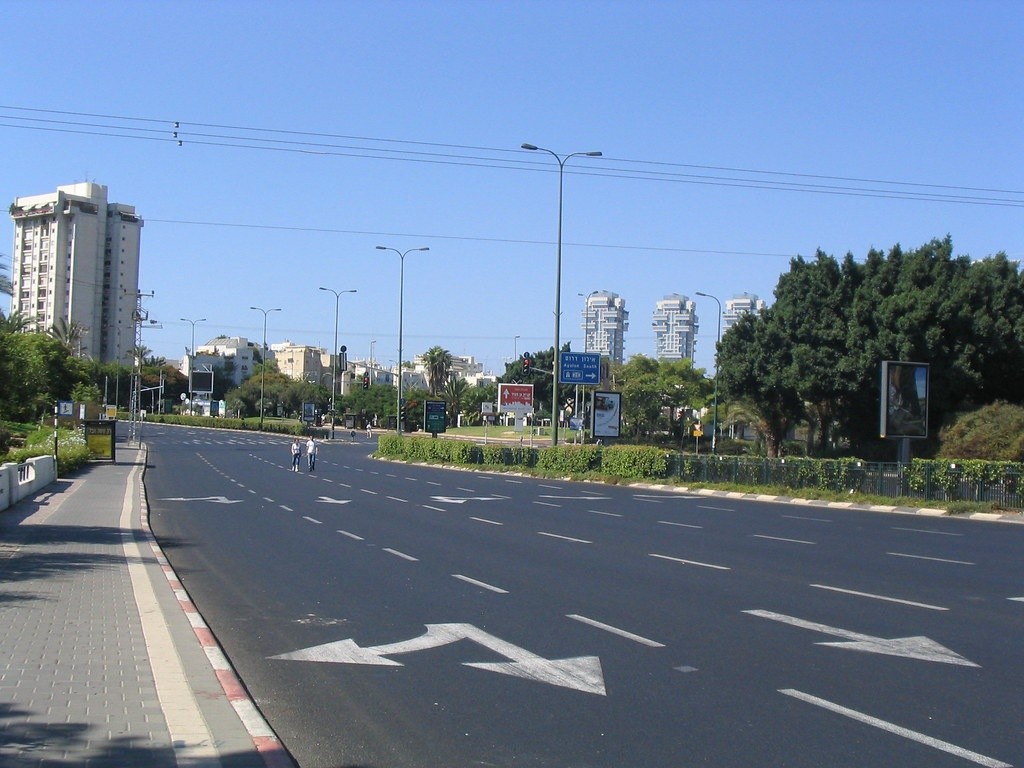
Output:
[559, 351, 601, 386]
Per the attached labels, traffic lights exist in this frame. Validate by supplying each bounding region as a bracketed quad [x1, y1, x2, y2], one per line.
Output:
[522, 357, 531, 376]
[364, 376, 371, 389]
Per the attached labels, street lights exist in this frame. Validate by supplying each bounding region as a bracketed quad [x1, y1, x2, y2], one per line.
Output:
[376, 245, 431, 438]
[250, 306, 282, 432]
[317, 286, 357, 440]
[695, 290, 722, 459]
[180, 318, 207, 418]
[520, 141, 603, 454]
[577, 291, 600, 443]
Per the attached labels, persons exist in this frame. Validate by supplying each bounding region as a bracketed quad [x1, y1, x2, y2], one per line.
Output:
[290, 438, 302, 472]
[365, 421, 372, 439]
[305, 434, 318, 471]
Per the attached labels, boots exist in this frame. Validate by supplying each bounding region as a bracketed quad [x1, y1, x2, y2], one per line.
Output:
[292, 466, 294, 471]
[295, 465, 298, 472]
